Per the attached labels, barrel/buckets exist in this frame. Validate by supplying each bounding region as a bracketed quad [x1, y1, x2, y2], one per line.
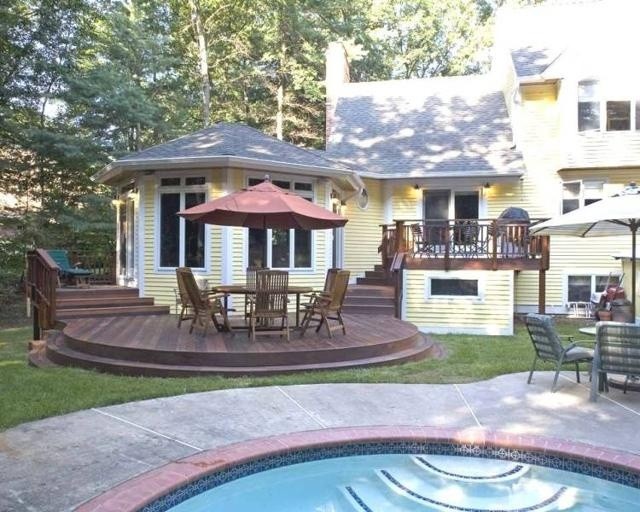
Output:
[611, 300, 632, 322]
[598, 309, 610, 321]
[607, 287, 624, 300]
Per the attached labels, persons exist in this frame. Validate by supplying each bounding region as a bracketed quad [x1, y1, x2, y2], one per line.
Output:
[528, 180, 640, 324]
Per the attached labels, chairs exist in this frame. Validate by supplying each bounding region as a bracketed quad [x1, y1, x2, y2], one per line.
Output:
[523, 310, 593, 392]
[405, 220, 497, 259]
[174, 259, 351, 341]
[589, 320, 640, 402]
[48, 249, 94, 289]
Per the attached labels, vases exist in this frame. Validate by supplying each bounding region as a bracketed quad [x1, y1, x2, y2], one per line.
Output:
[599, 311, 611, 321]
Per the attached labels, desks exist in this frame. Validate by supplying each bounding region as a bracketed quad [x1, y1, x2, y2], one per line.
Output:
[577, 326, 640, 393]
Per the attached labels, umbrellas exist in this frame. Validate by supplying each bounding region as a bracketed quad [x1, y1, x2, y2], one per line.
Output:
[175, 174, 348, 267]
